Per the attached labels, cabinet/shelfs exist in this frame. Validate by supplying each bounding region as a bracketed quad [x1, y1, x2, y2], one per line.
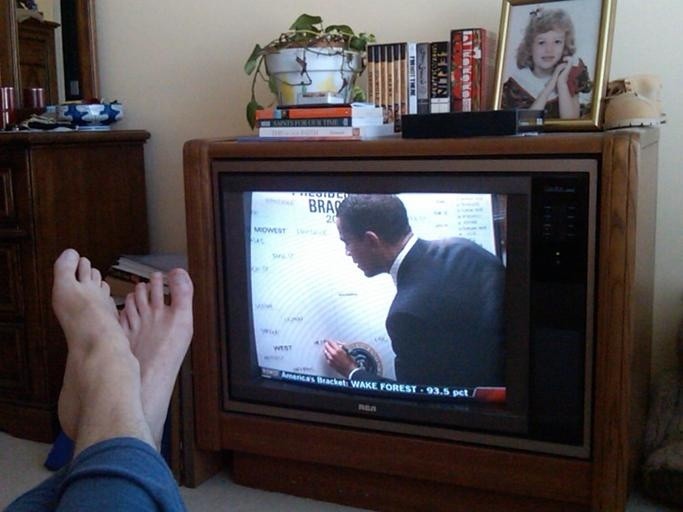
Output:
[18, 17, 62, 110]
[92, 278, 215, 488]
[0, 128, 156, 443]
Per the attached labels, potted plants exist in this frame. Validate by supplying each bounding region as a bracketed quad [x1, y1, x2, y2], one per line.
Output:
[243, 11, 377, 135]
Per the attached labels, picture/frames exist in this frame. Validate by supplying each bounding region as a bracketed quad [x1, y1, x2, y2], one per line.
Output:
[486, 1, 620, 131]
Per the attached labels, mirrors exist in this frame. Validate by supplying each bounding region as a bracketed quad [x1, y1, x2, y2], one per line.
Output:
[10, 1, 99, 111]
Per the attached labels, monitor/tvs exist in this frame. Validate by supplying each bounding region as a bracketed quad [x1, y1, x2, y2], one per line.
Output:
[209, 157, 595, 460]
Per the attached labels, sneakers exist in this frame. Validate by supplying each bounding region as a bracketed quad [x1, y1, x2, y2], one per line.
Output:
[604, 77, 660, 129]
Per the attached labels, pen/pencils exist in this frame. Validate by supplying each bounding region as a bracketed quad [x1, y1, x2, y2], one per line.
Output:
[337, 344, 354, 355]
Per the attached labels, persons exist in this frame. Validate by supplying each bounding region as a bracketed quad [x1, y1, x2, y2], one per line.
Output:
[0, 248, 193, 512]
[323, 193, 510, 398]
[502, 8, 593, 120]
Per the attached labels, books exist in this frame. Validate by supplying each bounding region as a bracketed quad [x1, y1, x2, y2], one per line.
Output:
[256, 28, 486, 141]
[103, 251, 188, 304]
